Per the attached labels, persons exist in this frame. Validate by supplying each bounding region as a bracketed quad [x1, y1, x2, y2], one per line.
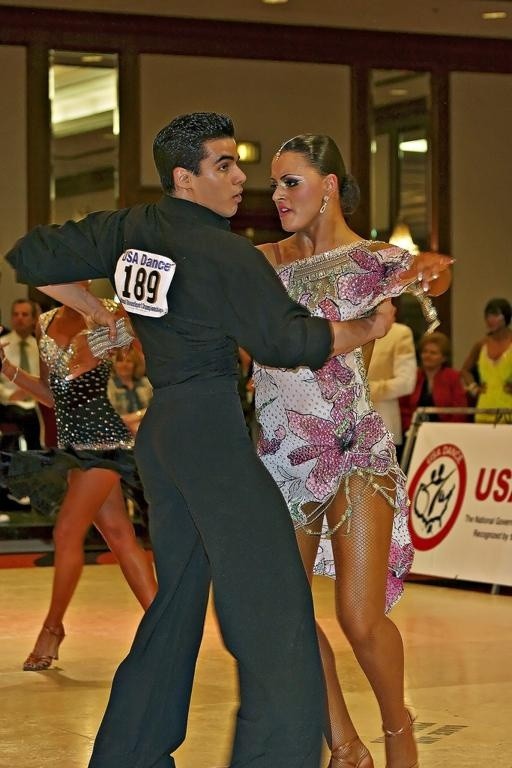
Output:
[0, 280, 161, 669]
[244, 132, 456, 767]
[4, 112, 332, 767]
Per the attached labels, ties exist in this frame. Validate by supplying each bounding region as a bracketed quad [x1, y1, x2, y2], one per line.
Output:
[16, 339, 30, 372]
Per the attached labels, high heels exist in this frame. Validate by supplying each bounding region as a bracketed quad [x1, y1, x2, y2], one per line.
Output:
[23, 622, 65, 670]
